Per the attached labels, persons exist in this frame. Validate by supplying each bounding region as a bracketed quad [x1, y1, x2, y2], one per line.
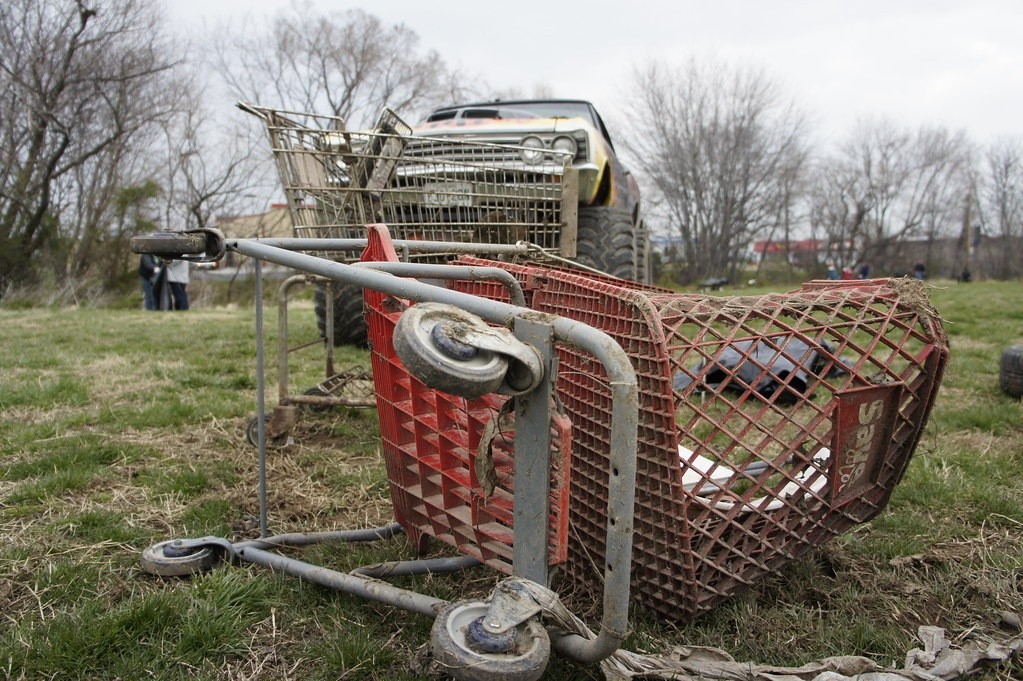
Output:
[914, 259, 925, 279]
[962, 265, 971, 282]
[859, 264, 870, 279]
[829, 261, 838, 280]
[139, 234, 189, 310]
[843, 265, 853, 279]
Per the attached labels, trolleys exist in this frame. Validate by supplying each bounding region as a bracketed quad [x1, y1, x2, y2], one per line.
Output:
[232, 101, 580, 453]
[126, 225, 949, 681]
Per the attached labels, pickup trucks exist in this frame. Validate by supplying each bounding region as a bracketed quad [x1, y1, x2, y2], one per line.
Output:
[312, 97, 653, 349]
